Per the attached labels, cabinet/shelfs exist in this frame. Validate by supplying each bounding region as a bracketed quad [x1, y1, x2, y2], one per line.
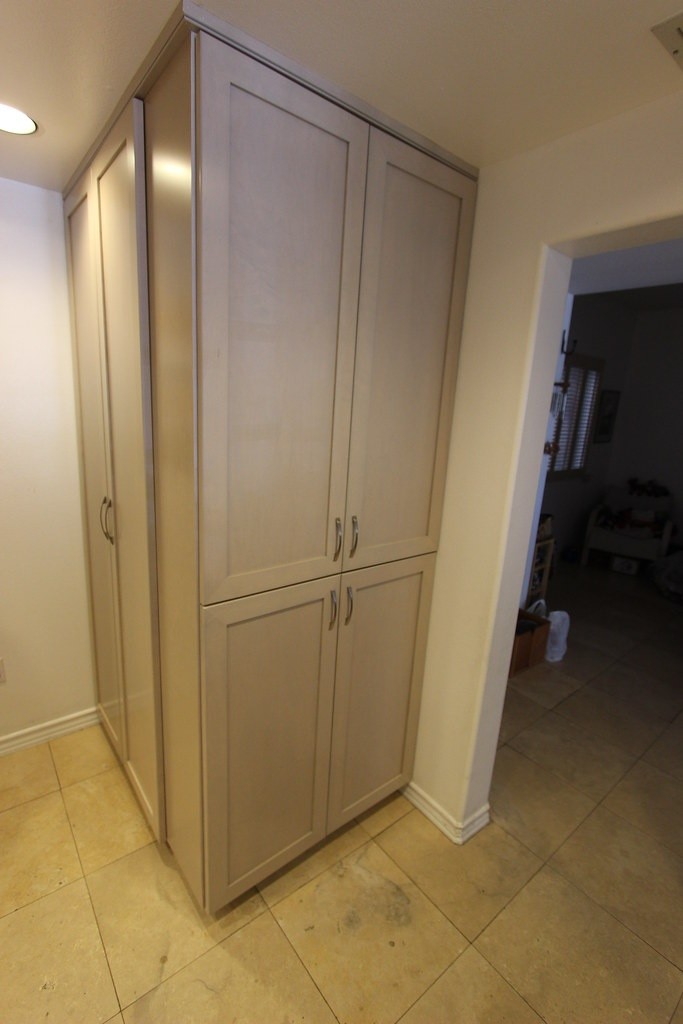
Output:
[64, 0, 482, 915]
[525, 537, 556, 611]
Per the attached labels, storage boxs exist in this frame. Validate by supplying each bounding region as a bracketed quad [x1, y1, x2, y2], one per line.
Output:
[509, 608, 551, 678]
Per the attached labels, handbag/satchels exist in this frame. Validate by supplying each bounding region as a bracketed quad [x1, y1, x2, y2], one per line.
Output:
[527, 599, 570, 662]
